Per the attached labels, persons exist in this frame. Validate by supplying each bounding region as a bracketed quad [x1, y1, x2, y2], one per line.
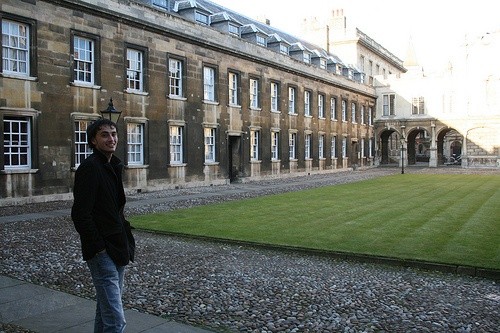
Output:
[71, 118, 137, 333]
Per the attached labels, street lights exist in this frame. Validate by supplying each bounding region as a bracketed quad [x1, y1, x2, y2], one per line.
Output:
[400, 134, 405, 174]
